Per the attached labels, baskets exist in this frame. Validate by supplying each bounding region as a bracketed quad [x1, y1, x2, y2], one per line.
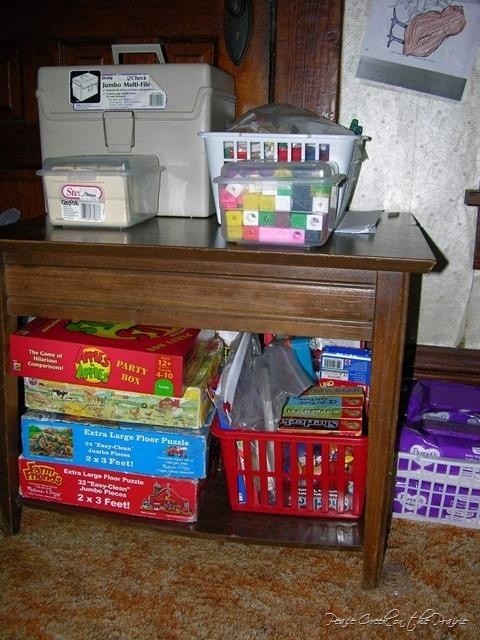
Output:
[391, 450, 479, 532]
[196, 126, 370, 235]
[209, 397, 369, 519]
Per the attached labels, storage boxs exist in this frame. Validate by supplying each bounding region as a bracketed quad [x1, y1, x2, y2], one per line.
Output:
[35, 44, 237, 232]
[213, 158, 347, 252]
[9, 317, 217, 523]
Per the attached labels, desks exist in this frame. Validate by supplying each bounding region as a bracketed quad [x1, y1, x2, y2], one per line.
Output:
[0, 211, 435, 589]
